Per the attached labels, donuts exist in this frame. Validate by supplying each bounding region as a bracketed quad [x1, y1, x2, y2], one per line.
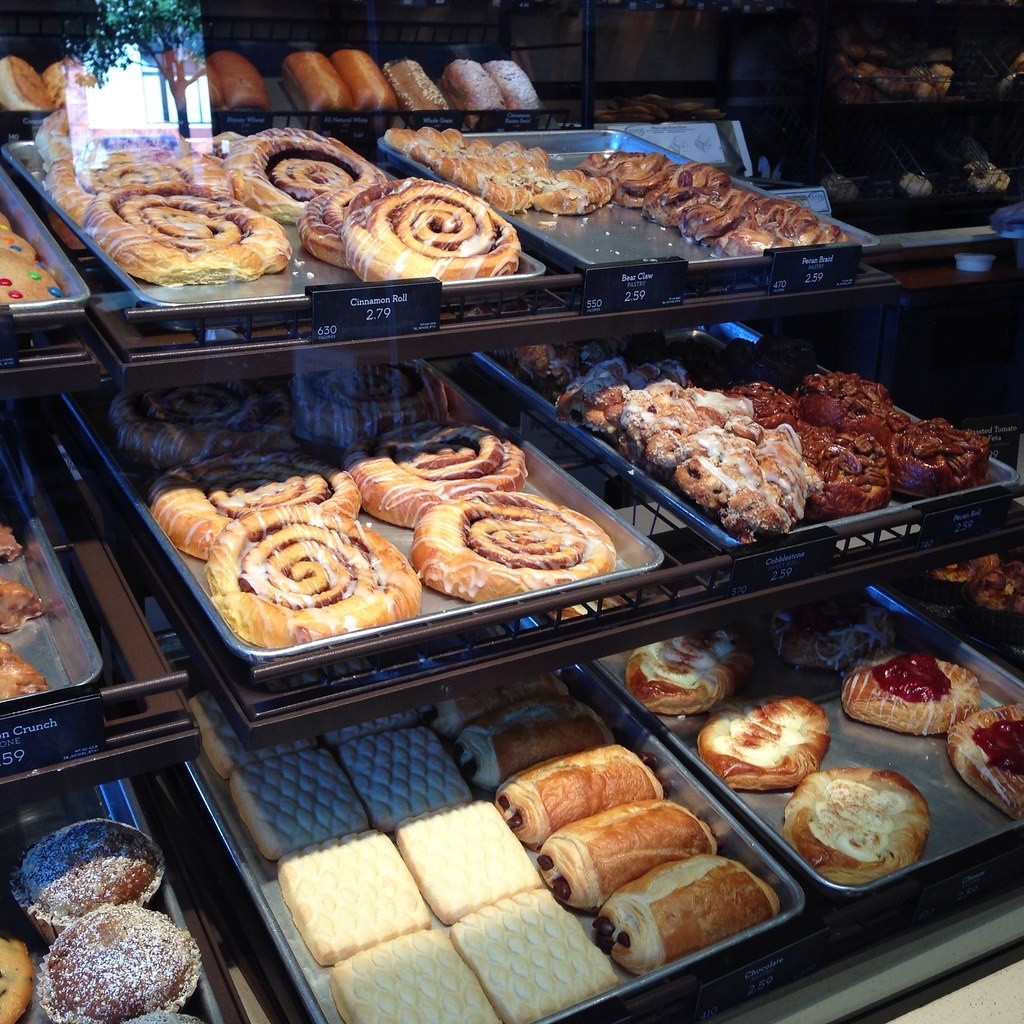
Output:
[819, 160, 1011, 202]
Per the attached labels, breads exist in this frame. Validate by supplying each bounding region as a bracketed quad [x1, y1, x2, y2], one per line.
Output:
[0, 322, 993, 710]
[0, 50, 858, 313]
[189, 547, 1024, 1024]
[775, 0, 1024, 104]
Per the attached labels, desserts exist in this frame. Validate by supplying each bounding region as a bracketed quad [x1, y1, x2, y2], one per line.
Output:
[0, 816, 209, 1024]
